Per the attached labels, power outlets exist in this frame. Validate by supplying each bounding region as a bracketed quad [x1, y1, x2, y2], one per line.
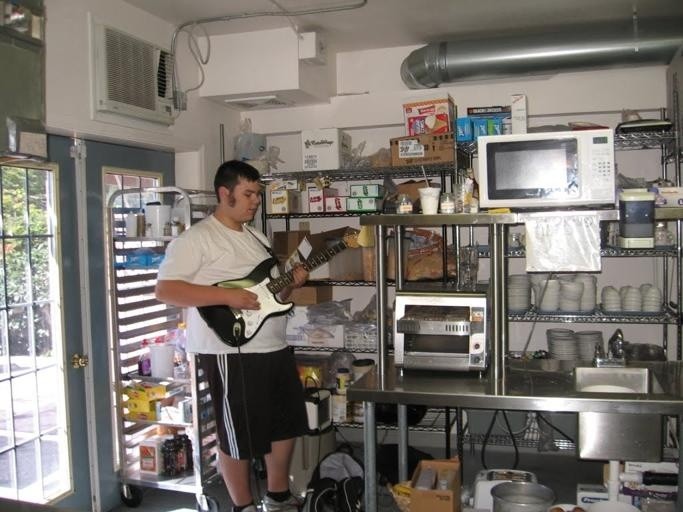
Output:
[173, 90, 188, 111]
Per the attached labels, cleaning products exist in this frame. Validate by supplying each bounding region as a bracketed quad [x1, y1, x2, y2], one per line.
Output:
[137, 340, 152, 375]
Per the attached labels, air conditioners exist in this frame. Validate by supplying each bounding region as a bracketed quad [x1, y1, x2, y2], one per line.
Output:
[95, 22, 176, 125]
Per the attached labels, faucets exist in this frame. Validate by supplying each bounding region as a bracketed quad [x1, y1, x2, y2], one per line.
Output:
[608, 328, 623, 357]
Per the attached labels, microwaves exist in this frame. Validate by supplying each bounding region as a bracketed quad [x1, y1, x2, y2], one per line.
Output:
[477, 129, 616, 209]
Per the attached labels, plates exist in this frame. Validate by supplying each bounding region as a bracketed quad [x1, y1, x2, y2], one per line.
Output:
[546, 328, 603, 363]
[507, 275, 531, 314]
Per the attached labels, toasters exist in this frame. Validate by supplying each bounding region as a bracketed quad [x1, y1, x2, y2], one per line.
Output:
[473, 470, 539, 510]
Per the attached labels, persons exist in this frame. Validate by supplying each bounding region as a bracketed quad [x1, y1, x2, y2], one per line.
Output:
[153, 158, 310, 512]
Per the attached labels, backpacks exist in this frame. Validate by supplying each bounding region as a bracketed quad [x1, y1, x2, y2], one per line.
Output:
[299, 443, 364, 511]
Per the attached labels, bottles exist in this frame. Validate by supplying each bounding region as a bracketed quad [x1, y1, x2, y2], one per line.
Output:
[138, 342, 152, 377]
[335, 359, 374, 396]
[509, 232, 522, 253]
[146, 224, 152, 238]
[164, 222, 171, 236]
[655, 223, 667, 247]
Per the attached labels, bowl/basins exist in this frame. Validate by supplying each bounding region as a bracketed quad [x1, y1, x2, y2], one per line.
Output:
[533, 272, 662, 313]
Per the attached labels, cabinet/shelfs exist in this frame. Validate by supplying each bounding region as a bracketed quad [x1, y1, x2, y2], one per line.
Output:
[103, 125, 682, 512]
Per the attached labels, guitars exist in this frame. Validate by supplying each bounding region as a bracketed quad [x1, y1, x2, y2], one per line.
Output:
[195, 233, 358, 348]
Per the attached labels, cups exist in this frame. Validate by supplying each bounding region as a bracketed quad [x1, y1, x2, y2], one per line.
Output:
[453, 183, 474, 213]
[458, 247, 478, 292]
[148, 343, 176, 378]
[420, 187, 441, 214]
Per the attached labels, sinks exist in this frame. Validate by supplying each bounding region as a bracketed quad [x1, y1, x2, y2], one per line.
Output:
[574, 362, 666, 462]
